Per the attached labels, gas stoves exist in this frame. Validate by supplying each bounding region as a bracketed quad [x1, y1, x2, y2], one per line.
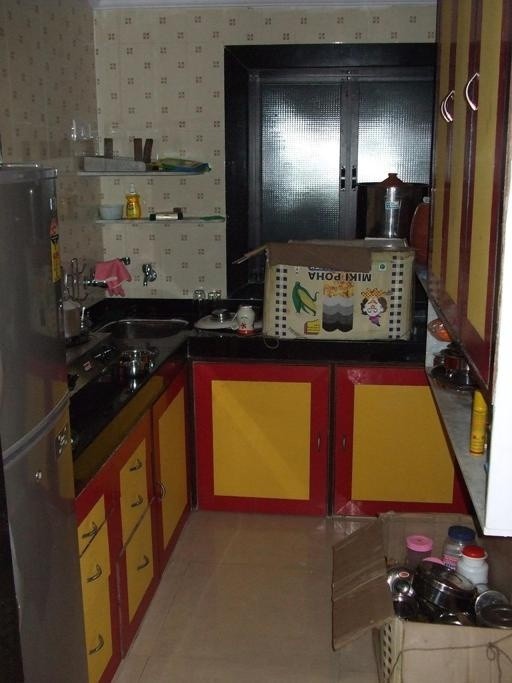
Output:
[65, 332, 117, 399]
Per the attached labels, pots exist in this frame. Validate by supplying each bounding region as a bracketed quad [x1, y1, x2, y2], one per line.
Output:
[428, 342, 479, 391]
[115, 347, 157, 380]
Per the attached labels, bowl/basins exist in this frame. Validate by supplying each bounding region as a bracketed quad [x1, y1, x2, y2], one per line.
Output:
[98, 203, 124, 220]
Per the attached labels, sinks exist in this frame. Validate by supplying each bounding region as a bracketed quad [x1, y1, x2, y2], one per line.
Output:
[92, 316, 189, 339]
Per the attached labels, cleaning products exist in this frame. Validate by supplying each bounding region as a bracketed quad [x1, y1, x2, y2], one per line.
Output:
[125, 184, 141, 218]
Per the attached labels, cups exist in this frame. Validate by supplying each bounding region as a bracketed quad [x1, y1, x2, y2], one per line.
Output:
[192, 289, 205, 306]
[208, 288, 221, 305]
[230, 305, 256, 336]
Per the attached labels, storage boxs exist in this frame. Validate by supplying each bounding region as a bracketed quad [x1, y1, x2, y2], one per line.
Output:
[231, 236, 421, 341]
[332, 508, 512, 682]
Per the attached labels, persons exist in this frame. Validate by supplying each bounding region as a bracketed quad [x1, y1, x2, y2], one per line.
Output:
[359, 296, 388, 315]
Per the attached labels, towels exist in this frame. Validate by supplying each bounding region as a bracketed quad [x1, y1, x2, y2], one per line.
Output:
[92, 258, 134, 298]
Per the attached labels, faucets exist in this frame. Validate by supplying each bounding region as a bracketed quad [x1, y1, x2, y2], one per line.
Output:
[142, 265, 157, 286]
[84, 256, 131, 288]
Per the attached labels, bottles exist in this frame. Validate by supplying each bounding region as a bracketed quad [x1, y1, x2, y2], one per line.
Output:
[126, 183, 142, 219]
[469, 389, 489, 455]
[404, 533, 433, 570]
[377, 172, 408, 237]
[455, 545, 489, 585]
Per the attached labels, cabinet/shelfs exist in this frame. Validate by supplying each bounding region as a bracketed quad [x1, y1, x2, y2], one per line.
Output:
[75, 410, 152, 683]
[78, 168, 230, 226]
[151, 360, 198, 571]
[185, 355, 470, 518]
[459, 0, 512, 390]
[429, 0, 473, 314]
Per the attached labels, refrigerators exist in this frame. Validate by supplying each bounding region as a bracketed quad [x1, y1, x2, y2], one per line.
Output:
[0, 161, 92, 682]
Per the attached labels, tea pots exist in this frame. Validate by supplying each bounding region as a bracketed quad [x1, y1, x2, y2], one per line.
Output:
[62, 291, 87, 338]
